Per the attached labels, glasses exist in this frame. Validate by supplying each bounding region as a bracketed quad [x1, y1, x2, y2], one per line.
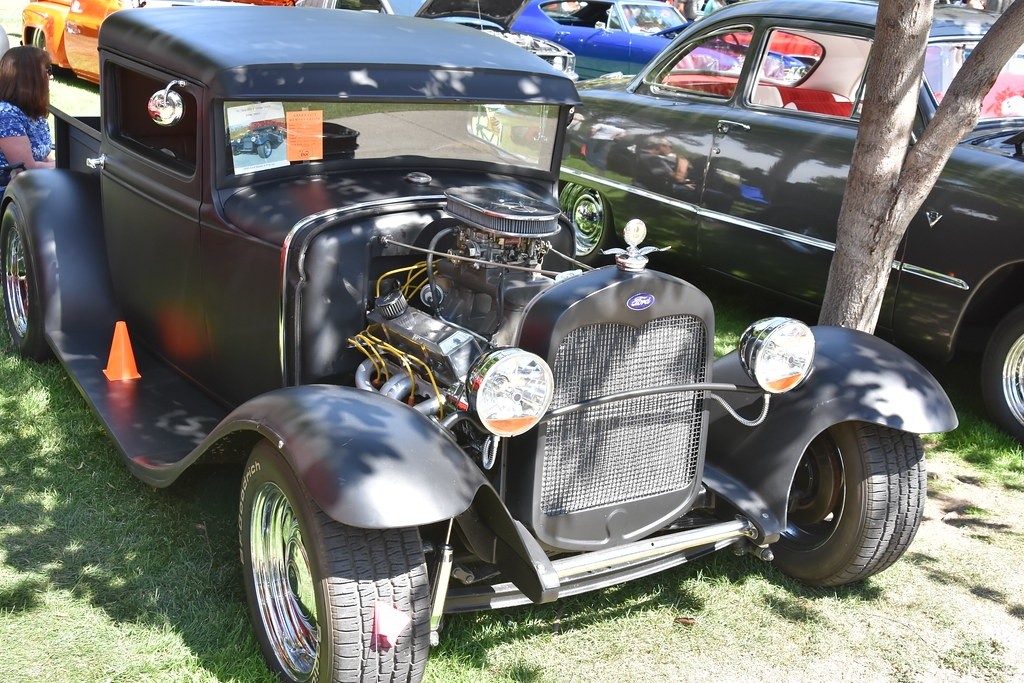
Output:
[45, 65, 52, 75]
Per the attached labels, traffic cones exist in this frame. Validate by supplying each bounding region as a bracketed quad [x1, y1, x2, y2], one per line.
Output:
[102, 320, 143, 382]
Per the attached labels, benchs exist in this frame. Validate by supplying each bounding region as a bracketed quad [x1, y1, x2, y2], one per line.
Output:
[675, 80, 853, 120]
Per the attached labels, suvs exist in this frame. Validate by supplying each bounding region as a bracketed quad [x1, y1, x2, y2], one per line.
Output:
[230, 125, 285, 158]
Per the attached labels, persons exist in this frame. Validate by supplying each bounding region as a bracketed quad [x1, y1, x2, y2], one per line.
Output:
[0, 46, 56, 206]
[662, 0, 740, 24]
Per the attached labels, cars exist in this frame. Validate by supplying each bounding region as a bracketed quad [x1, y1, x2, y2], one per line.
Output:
[659, 27, 1024, 121]
[557, 0, 1024, 443]
[1, 0, 798, 307]
[0, 5, 962, 683]
